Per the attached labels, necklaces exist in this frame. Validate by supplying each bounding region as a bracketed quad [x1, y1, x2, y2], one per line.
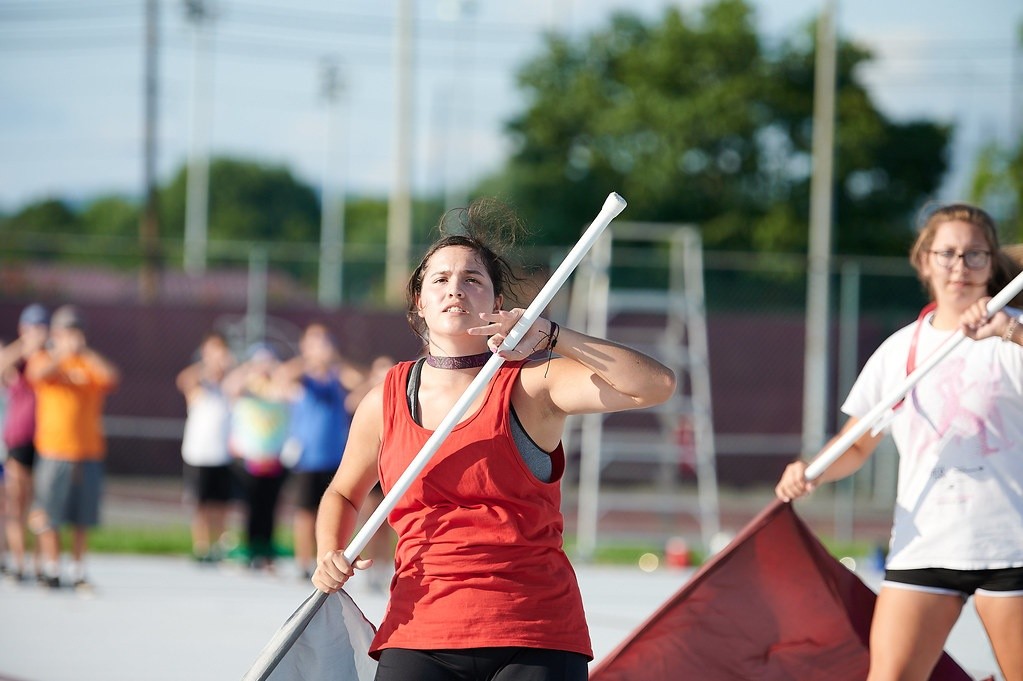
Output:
[426, 350, 493, 370]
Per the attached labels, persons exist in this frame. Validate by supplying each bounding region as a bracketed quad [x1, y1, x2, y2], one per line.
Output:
[774, 204, 1023, 681]
[311, 194, 675, 681]
[174, 321, 395, 584]
[0, 303, 121, 591]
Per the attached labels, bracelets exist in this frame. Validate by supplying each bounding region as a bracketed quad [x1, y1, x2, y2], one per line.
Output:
[530, 321, 560, 378]
[1001, 317, 1019, 343]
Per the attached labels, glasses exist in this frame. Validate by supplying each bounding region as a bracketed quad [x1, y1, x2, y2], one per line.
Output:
[927, 249, 991, 270]
